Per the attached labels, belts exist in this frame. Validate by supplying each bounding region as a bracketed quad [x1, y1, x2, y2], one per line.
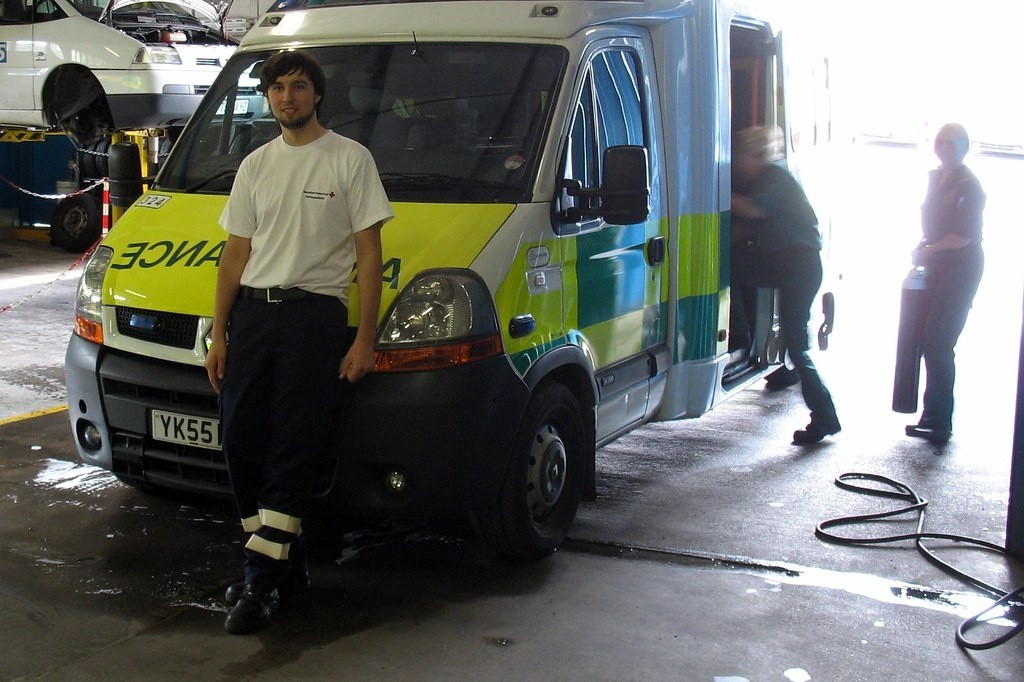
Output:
[237, 285, 327, 304]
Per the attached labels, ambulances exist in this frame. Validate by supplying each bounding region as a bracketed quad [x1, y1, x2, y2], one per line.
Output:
[64, 0, 834, 566]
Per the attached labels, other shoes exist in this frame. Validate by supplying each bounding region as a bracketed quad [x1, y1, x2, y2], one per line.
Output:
[905, 421, 952, 439]
[794, 409, 841, 443]
[766, 367, 801, 391]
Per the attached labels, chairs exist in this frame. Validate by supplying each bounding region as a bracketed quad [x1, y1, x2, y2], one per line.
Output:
[326, 78, 383, 150]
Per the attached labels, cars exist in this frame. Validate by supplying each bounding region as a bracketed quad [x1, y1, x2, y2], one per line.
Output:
[0, 0, 272, 130]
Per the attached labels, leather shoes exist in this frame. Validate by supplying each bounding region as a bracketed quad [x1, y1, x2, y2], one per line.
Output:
[224, 584, 280, 635]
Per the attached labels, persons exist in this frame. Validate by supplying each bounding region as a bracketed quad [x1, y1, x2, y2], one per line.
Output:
[203, 52, 393, 634]
[905, 121, 986, 442]
[728, 121, 840, 446]
